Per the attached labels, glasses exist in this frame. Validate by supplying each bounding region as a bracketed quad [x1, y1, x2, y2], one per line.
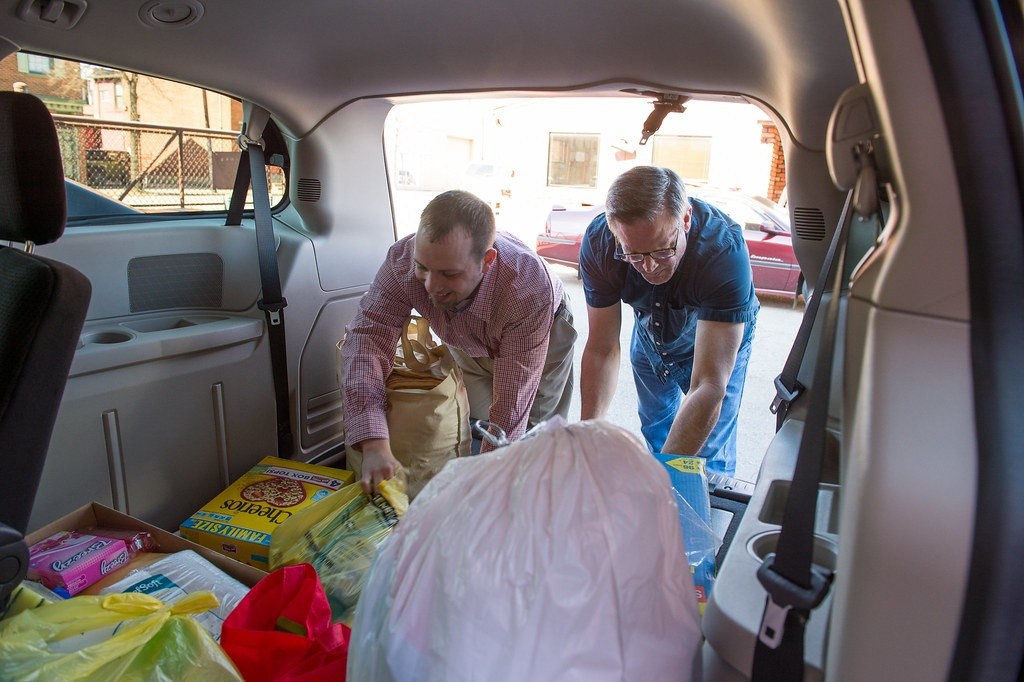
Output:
[614, 222, 680, 263]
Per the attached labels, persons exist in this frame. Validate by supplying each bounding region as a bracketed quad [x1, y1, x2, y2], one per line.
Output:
[337, 190, 578, 501]
[579, 165, 761, 479]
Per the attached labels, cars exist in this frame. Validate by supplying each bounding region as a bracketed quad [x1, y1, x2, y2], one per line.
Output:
[536, 179, 811, 308]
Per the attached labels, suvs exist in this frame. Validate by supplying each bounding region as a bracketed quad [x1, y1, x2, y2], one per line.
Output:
[86, 150, 133, 188]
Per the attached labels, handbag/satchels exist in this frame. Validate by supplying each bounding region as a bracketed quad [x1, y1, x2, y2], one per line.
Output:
[335, 311, 474, 498]
[219, 562, 350, 681]
